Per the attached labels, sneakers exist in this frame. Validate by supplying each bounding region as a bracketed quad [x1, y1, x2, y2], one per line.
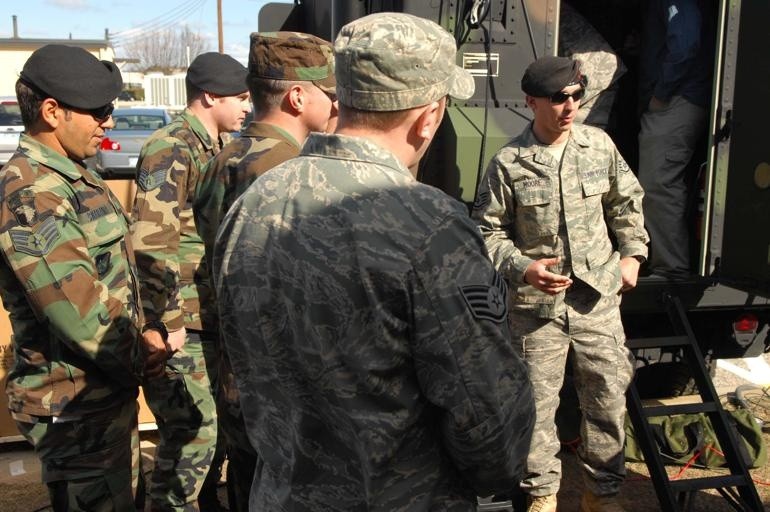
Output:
[526, 491, 559, 512]
[580, 485, 628, 512]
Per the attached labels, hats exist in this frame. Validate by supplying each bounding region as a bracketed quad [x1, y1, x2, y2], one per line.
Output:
[520, 55, 583, 98]
[248, 30, 341, 96]
[333, 12, 476, 114]
[21, 44, 125, 109]
[184, 51, 254, 99]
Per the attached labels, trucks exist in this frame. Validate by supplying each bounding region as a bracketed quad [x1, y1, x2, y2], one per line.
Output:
[255, 0, 770, 399]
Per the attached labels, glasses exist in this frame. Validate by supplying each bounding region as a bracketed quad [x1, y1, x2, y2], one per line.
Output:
[57, 103, 114, 120]
[549, 87, 586, 104]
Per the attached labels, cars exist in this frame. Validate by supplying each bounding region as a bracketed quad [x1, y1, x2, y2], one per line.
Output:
[1, 94, 24, 168]
[93, 108, 173, 177]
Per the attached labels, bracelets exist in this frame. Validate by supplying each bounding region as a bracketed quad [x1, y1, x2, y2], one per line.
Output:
[143, 320, 168, 339]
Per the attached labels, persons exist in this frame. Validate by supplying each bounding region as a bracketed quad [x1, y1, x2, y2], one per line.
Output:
[0, 45, 168, 512]
[469, 57, 652, 512]
[211, 13, 536, 511]
[191, 31, 339, 512]
[128, 52, 252, 512]
[560, 0, 629, 131]
[637, 0, 716, 280]
